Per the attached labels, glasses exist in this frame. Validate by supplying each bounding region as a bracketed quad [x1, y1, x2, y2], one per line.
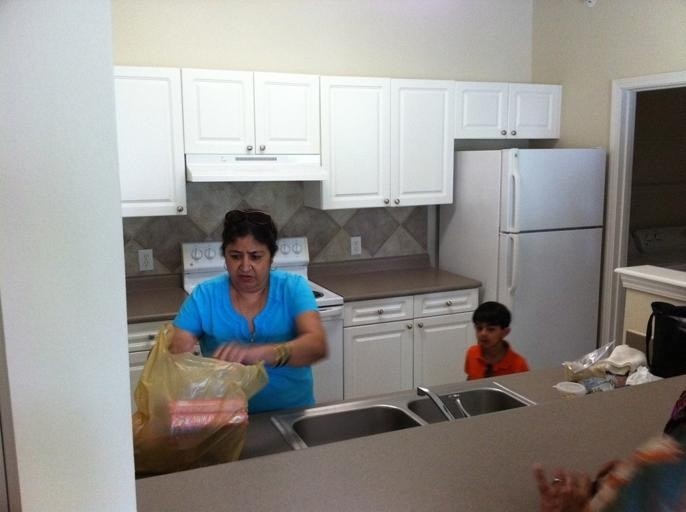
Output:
[226, 209, 270, 227]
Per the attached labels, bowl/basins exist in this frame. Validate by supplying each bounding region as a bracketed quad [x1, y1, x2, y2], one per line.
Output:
[554, 381, 589, 399]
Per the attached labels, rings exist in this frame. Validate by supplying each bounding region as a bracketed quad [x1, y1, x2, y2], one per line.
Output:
[551, 478, 566, 485]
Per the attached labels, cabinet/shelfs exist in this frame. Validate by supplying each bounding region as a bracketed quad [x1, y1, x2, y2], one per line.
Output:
[343, 287, 479, 400]
[180, 66, 321, 182]
[128, 320, 203, 410]
[322, 75, 453, 212]
[454, 82, 562, 140]
[113, 65, 187, 218]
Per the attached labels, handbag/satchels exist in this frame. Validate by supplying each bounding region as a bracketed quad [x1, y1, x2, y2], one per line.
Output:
[643, 301, 685, 377]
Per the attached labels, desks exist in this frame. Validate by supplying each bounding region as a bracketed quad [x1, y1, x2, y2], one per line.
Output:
[613, 265, 686, 347]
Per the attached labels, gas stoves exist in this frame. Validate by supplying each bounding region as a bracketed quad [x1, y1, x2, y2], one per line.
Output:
[182, 279, 344, 308]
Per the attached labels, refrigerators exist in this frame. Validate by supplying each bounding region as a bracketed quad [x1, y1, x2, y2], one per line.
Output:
[436, 146, 608, 386]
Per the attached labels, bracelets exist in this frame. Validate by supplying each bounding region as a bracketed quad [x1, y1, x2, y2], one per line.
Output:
[273, 339, 293, 370]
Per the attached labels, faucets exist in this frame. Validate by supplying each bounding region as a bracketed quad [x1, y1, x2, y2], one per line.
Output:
[416, 385, 457, 421]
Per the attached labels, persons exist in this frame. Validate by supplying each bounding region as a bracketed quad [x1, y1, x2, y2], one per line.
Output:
[531, 436, 686, 512]
[461, 301, 528, 381]
[163, 210, 329, 416]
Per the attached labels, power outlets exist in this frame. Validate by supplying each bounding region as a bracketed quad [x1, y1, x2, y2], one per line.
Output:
[351, 236, 362, 255]
[138, 249, 153, 271]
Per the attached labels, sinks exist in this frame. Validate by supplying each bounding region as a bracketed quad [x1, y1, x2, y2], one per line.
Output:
[408, 381, 537, 426]
[269, 398, 421, 449]
